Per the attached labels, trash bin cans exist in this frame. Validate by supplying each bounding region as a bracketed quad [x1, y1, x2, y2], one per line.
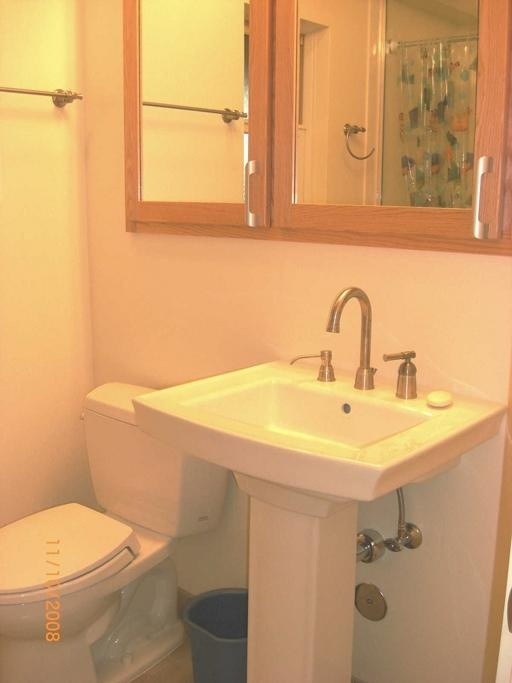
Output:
[183, 587, 248, 683]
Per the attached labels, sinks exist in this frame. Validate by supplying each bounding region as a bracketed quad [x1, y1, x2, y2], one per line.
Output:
[129, 358, 507, 502]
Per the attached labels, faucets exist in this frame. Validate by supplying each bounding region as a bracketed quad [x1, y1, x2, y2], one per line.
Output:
[327, 286, 378, 390]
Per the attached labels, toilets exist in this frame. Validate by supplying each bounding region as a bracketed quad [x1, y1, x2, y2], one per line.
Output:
[1, 381, 230, 682]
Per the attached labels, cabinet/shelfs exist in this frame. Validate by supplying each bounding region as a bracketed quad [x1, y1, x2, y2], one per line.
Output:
[271, 2, 512, 255]
[123, 1, 273, 240]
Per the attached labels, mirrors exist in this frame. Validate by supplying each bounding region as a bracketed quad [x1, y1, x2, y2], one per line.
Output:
[139, 3, 246, 205]
[295, 3, 478, 207]
